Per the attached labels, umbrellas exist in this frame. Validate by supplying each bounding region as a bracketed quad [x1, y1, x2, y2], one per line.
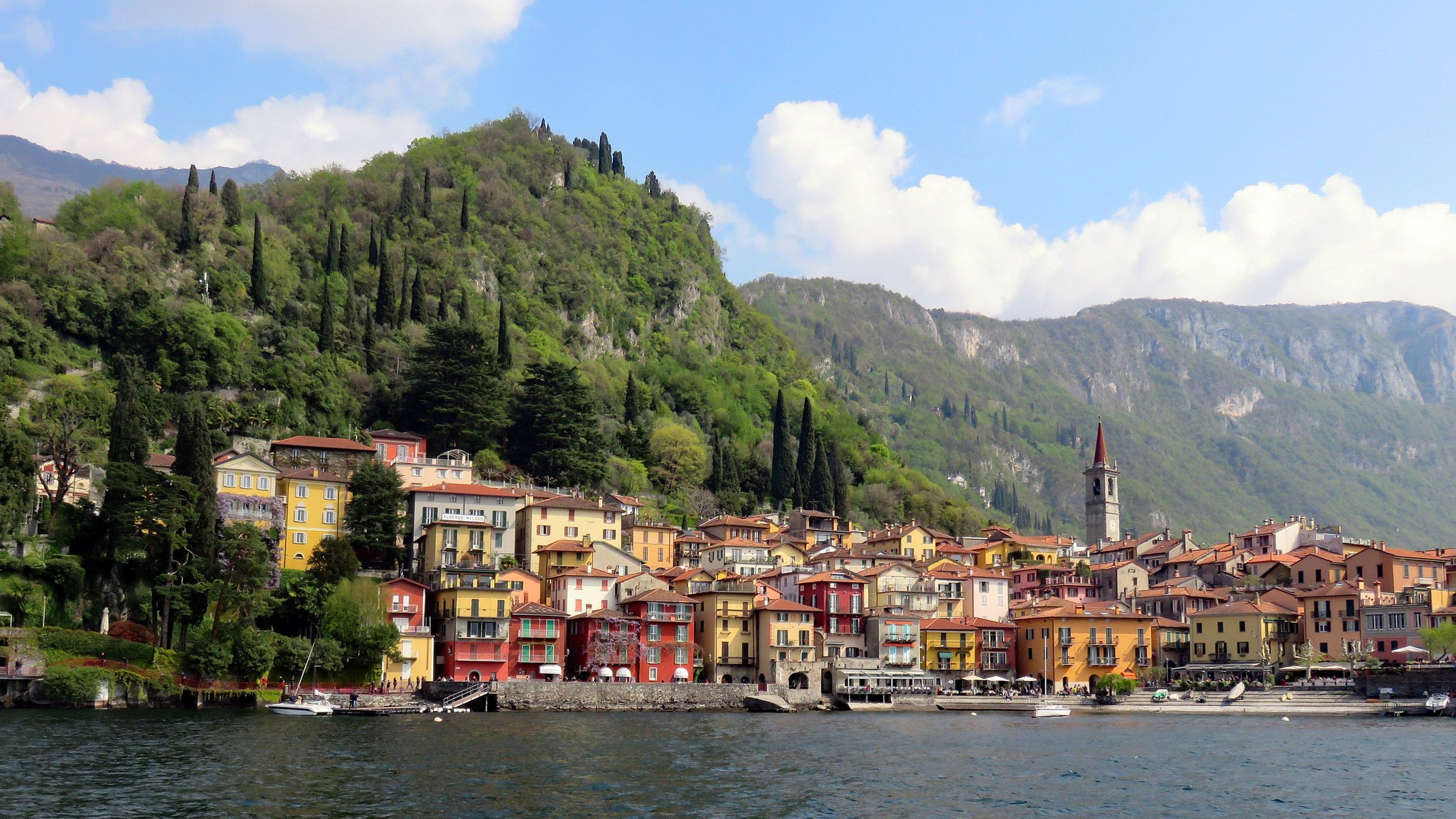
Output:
[1391, 645, 1430, 661]
[100, 607, 109, 635]
[960, 674, 1037, 687]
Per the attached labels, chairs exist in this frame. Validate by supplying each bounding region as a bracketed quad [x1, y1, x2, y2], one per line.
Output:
[844, 686, 932, 694]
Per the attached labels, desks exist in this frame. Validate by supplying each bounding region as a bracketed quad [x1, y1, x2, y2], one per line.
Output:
[943, 690, 995, 695]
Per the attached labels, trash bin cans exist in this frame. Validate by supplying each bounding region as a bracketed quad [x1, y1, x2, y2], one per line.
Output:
[283, 685, 289, 695]
[1379, 694, 1390, 700]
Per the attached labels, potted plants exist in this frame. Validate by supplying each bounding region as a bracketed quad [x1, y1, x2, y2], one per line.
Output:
[1014, 691, 1018, 697]
[885, 655, 889, 665]
[1172, 674, 1275, 688]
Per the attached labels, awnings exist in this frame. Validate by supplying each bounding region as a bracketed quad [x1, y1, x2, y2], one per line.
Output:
[597, 667, 631, 678]
[938, 652, 953, 659]
[1280, 662, 1385, 670]
[674, 667, 688, 678]
[538, 664, 562, 674]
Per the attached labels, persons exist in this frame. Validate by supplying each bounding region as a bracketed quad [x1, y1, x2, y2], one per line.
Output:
[368, 675, 426, 695]
[526, 676, 530, 682]
[938, 686, 952, 695]
[1142, 675, 1251, 687]
[591, 675, 603, 682]
[245, 675, 267, 690]
[98, 652, 104, 667]
[279, 676, 295, 691]
[564, 675, 577, 681]
[15, 658, 24, 675]
[962, 683, 1042, 699]
[123, 656, 128, 670]
[662, 678, 665, 683]
[437, 675, 498, 682]
[1300, 676, 1336, 684]
[1059, 684, 1088, 699]
[181, 672, 187, 686]
[349, 692, 359, 709]
[296, 695, 303, 704]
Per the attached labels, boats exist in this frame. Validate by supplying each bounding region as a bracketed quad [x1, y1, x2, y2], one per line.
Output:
[1183, 689, 1194, 699]
[1280, 693, 1287, 701]
[1227, 681, 1245, 701]
[1426, 693, 1449, 713]
[1287, 692, 1293, 700]
[1200, 694, 1208, 702]
[1153, 689, 1168, 702]
[1170, 694, 1179, 700]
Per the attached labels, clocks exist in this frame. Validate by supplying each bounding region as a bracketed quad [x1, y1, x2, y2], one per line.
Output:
[1109, 519, 1116, 532]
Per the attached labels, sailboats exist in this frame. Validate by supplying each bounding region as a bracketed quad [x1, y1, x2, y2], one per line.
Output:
[264, 626, 334, 716]
[1034, 635, 1071, 717]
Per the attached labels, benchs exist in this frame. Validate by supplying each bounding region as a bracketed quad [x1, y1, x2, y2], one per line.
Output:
[1056, 692, 1069, 698]
